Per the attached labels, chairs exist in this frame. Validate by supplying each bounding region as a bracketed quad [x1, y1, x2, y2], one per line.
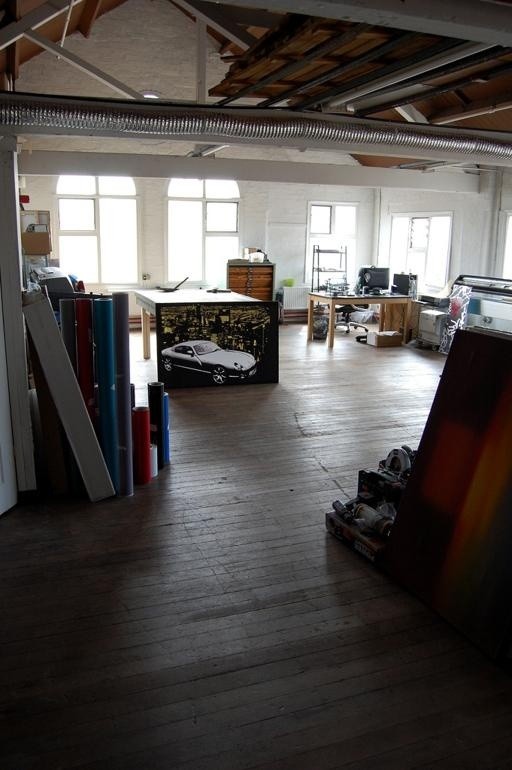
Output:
[332, 286, 371, 333]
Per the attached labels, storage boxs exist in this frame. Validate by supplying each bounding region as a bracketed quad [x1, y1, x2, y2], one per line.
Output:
[366, 330, 402, 347]
[24, 231, 52, 254]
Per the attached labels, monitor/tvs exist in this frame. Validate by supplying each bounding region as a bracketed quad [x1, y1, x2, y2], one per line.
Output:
[363, 267, 389, 296]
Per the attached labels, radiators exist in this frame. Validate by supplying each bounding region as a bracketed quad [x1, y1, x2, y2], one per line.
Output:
[109, 289, 151, 318]
[283, 288, 310, 308]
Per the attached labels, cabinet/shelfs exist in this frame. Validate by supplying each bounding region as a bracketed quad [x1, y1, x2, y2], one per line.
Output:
[227, 259, 275, 301]
[18, 211, 50, 289]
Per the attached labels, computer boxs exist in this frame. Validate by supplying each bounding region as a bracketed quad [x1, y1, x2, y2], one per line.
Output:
[394, 273, 418, 296]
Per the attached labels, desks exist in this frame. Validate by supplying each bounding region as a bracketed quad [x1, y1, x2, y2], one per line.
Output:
[306, 288, 411, 349]
[134, 289, 262, 360]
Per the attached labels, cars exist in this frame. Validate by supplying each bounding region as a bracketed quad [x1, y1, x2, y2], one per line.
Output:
[162, 339, 258, 385]
